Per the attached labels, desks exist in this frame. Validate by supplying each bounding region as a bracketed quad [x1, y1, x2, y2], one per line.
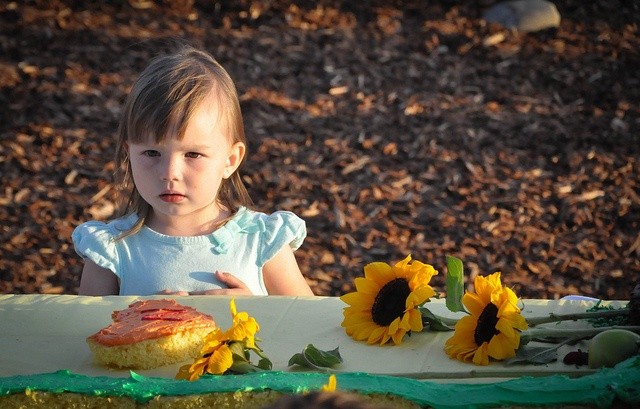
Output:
[0, 293, 637, 407]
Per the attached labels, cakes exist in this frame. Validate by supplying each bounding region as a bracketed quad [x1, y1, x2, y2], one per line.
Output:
[86, 300, 217, 369]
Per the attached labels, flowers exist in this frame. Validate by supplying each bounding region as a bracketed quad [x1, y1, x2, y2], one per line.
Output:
[177, 299, 343, 394]
[338, 254, 638, 364]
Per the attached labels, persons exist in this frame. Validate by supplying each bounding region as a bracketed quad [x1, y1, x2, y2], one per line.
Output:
[72, 48, 314, 295]
